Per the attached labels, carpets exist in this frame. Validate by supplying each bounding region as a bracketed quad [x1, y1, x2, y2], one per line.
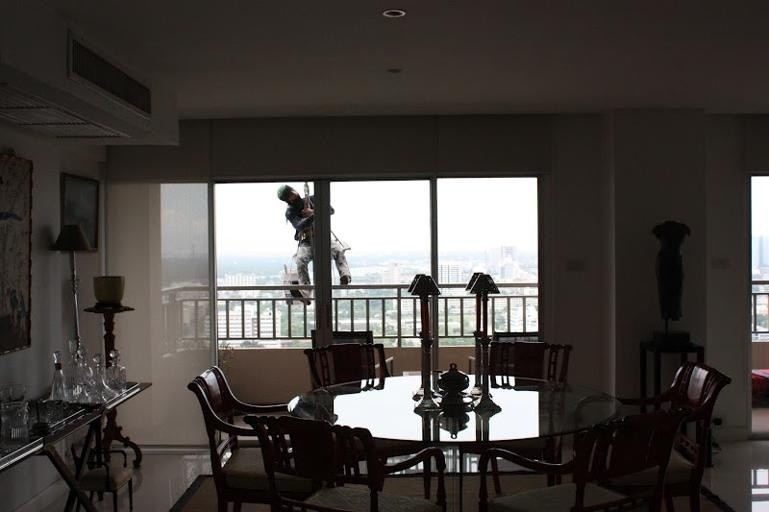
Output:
[169, 469, 637, 512]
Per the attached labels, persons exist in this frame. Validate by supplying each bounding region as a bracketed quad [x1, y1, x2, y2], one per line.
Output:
[277, 184, 353, 305]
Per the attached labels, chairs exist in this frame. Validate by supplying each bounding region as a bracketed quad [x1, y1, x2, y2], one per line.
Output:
[187, 365, 330, 511]
[478, 414, 649, 512]
[490, 341, 574, 486]
[613, 362, 732, 512]
[639, 340, 706, 416]
[304, 343, 387, 389]
[311, 328, 394, 377]
[241, 415, 447, 511]
[467, 330, 544, 376]
[71, 441, 135, 512]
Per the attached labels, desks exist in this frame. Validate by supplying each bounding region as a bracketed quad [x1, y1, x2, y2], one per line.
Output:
[287, 370, 623, 501]
[0, 381, 153, 512]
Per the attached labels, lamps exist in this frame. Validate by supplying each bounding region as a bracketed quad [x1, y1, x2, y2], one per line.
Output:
[51, 221, 94, 357]
[410, 276, 445, 413]
[408, 275, 444, 398]
[470, 274, 500, 412]
[85, 276, 135, 370]
[464, 273, 494, 398]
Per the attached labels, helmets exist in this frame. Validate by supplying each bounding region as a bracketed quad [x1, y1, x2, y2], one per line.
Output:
[277, 184, 302, 206]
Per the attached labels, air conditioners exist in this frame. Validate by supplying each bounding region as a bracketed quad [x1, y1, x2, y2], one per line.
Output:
[66, 33, 156, 124]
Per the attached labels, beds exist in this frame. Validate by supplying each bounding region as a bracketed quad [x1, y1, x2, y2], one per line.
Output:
[752, 369, 769, 404]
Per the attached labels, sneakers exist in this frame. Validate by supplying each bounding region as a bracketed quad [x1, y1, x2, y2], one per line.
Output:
[338, 274, 354, 288]
[289, 284, 312, 307]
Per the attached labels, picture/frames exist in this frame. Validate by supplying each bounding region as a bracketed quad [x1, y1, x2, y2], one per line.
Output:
[0, 150, 34, 356]
[59, 169, 101, 254]
